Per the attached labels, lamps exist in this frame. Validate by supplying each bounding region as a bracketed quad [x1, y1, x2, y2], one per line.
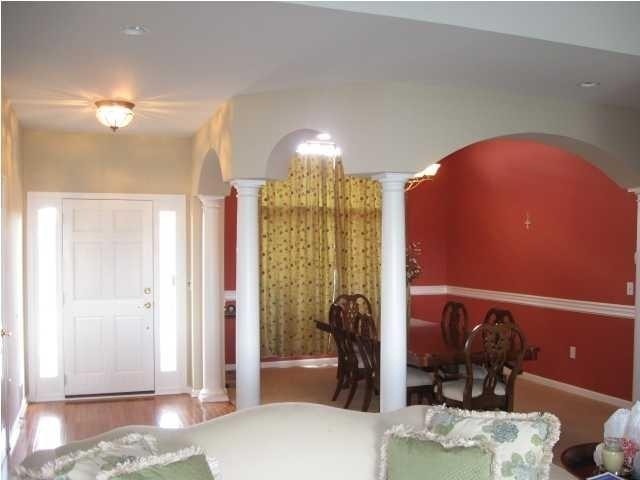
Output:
[403, 162, 441, 193]
[95, 98, 137, 132]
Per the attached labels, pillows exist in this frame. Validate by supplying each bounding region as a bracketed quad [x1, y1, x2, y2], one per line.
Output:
[36, 430, 155, 480]
[367, 423, 500, 480]
[95, 444, 222, 480]
[422, 401, 564, 480]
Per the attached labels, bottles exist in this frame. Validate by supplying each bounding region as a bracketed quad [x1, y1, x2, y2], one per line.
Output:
[601, 439, 625, 472]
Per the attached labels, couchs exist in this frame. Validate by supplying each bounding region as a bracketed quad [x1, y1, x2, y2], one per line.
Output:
[15, 394, 577, 480]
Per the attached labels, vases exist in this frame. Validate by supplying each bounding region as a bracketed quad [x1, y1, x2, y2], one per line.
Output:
[600, 438, 627, 473]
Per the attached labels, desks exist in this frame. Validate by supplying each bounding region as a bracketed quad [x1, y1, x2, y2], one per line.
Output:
[560, 437, 640, 480]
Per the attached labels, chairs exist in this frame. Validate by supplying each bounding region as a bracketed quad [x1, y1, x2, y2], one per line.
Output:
[310, 285, 541, 414]
